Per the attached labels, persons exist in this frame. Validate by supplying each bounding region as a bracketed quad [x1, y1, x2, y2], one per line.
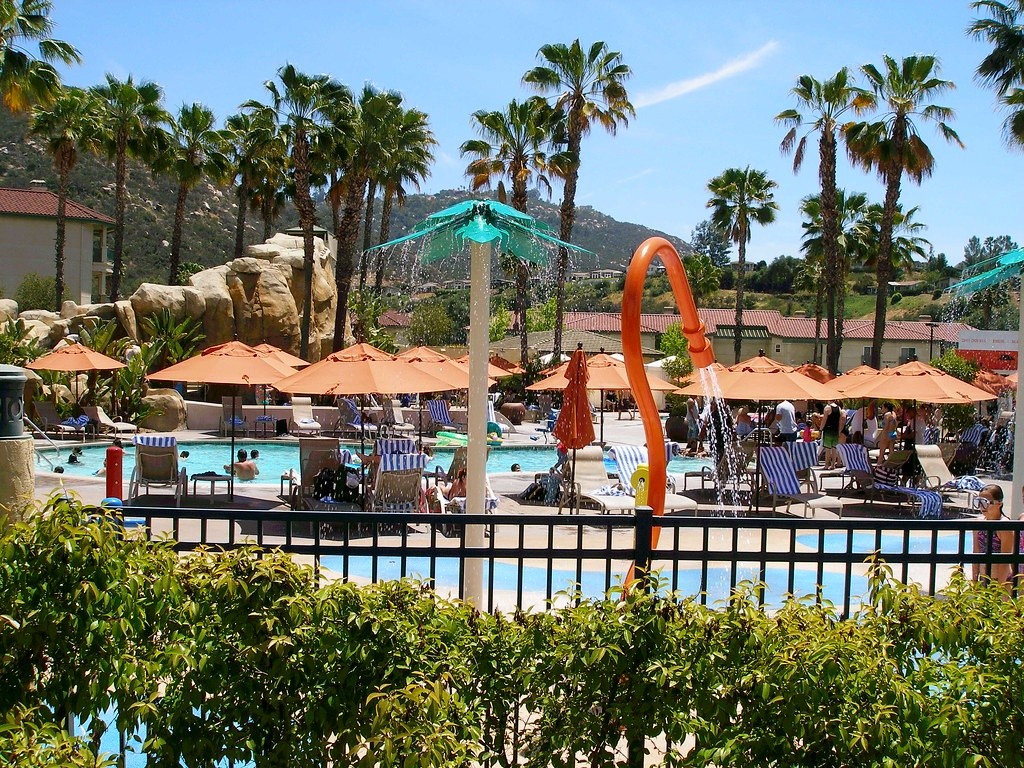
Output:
[684, 396, 874, 471]
[356, 445, 403, 489]
[180, 451, 189, 458]
[511, 463, 520, 472]
[439, 467, 466, 501]
[54, 467, 64, 473]
[113, 438, 125, 454]
[972, 483, 1013, 602]
[93, 459, 106, 477]
[224, 449, 259, 480]
[899, 407, 926, 488]
[1016, 487, 1024, 596]
[68, 447, 80, 462]
[873, 402, 897, 464]
[251, 450, 259, 458]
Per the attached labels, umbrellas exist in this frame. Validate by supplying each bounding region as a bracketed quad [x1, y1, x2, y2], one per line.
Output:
[525, 347, 999, 449]
[673, 349, 847, 512]
[253, 338, 311, 436]
[270, 334, 527, 506]
[24, 339, 127, 403]
[551, 341, 595, 515]
[144, 333, 300, 501]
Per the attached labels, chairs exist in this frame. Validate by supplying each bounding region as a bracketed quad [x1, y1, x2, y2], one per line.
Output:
[31, 400, 1015, 519]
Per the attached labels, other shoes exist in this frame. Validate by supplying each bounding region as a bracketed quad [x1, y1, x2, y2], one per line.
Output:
[836, 464, 843, 468]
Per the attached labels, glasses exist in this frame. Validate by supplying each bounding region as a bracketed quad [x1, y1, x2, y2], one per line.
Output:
[972, 496, 990, 509]
